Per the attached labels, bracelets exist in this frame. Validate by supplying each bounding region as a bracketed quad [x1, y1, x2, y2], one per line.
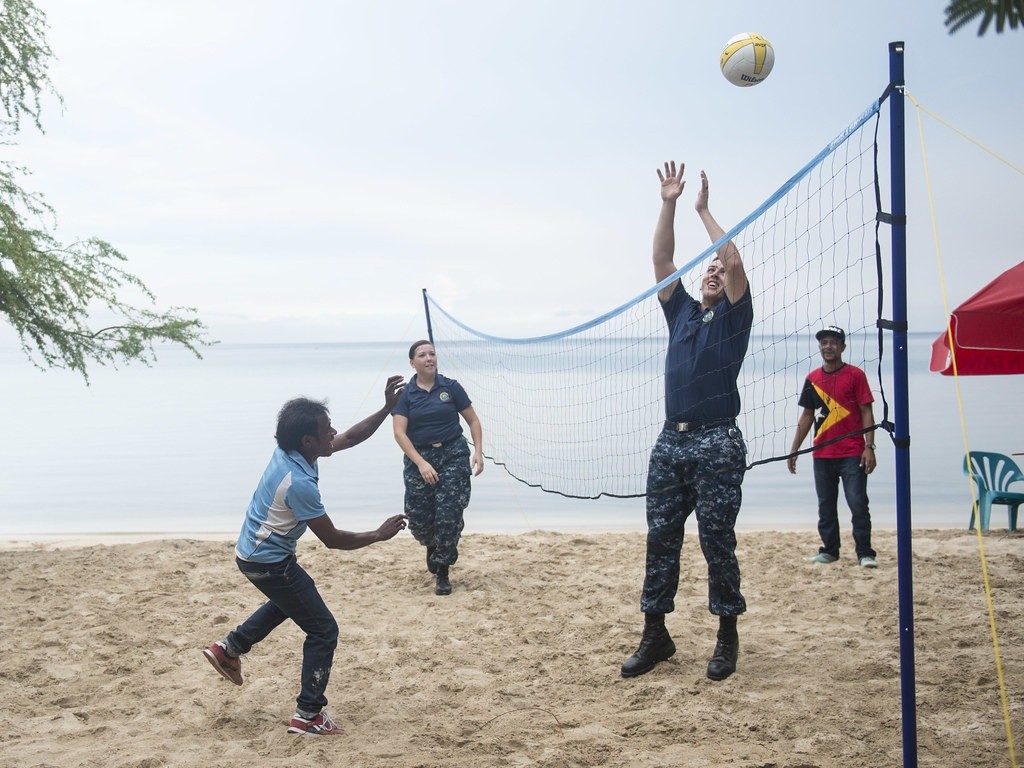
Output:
[865, 444, 876, 449]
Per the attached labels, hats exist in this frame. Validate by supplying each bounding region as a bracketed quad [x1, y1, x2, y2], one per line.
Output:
[816, 326, 845, 343]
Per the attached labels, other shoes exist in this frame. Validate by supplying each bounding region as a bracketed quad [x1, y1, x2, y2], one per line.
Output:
[803, 552, 838, 564]
[861, 554, 878, 567]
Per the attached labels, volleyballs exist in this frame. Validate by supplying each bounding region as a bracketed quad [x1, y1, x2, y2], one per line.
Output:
[721, 31, 775, 88]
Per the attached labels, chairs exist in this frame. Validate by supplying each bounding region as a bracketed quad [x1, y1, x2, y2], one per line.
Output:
[963, 451, 1024, 532]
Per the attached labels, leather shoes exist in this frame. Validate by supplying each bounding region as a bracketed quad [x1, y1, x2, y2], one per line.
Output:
[436, 568, 452, 596]
[426, 547, 437, 574]
[414, 435, 461, 449]
[707, 630, 739, 680]
[621, 623, 676, 676]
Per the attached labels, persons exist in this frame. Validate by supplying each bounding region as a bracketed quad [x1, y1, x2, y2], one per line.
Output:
[203, 375, 408, 737]
[392, 339, 485, 595]
[788, 327, 876, 568]
[620, 159, 754, 682]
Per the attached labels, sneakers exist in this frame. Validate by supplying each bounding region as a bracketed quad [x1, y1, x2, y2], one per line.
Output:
[287, 709, 346, 736]
[202, 641, 243, 686]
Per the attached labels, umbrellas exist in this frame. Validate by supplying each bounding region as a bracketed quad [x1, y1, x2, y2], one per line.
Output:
[929, 260, 1024, 375]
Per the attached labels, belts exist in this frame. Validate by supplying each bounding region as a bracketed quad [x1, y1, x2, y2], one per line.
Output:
[664, 418, 735, 432]
